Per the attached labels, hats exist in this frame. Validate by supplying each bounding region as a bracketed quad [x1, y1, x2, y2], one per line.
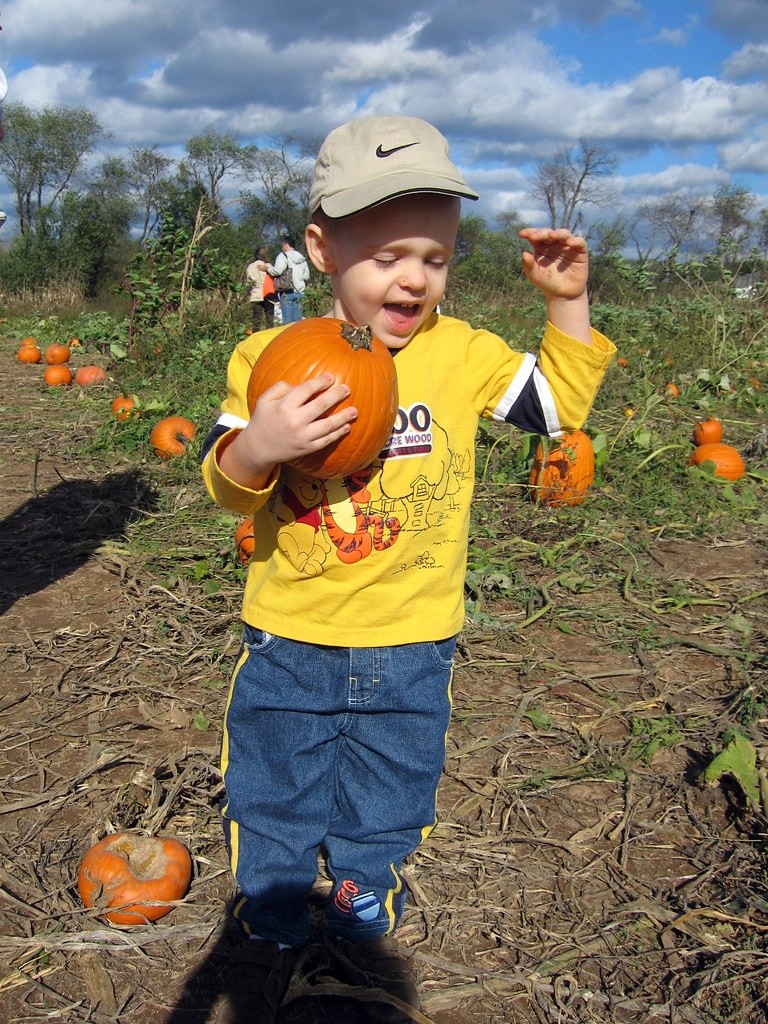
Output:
[308, 117, 479, 224]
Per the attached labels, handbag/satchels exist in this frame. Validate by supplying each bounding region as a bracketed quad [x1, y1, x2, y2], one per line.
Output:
[273, 252, 294, 292]
[263, 273, 277, 298]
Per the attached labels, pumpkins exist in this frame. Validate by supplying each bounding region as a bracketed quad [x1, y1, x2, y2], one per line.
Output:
[246, 316, 399, 480]
[18, 336, 195, 459]
[528, 356, 761, 507]
[235, 515, 256, 556]
[79, 831, 191, 925]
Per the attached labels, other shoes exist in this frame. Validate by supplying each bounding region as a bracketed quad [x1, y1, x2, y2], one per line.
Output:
[324, 928, 420, 1024]
[216, 936, 297, 1024]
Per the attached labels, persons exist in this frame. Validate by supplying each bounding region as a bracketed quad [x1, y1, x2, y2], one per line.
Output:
[190, 115, 619, 1010]
[245, 238, 311, 333]
